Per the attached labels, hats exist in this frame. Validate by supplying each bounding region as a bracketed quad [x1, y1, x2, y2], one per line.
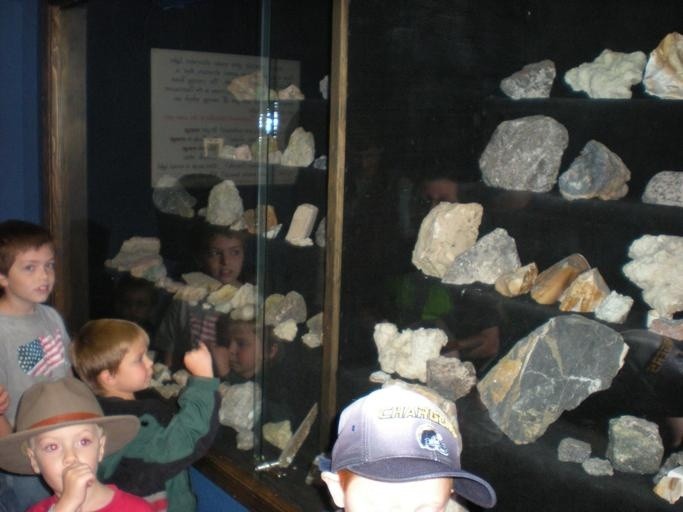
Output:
[0, 380, 140, 476]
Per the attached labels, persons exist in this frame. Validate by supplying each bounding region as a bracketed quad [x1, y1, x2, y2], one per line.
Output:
[368, 164, 465, 340]
[0, 378, 156, 511]
[578, 327, 683, 451]
[211, 314, 304, 464]
[0, 385, 15, 437]
[115, 276, 160, 358]
[0, 220, 79, 430]
[448, 188, 557, 371]
[69, 317, 220, 512]
[155, 222, 253, 367]
